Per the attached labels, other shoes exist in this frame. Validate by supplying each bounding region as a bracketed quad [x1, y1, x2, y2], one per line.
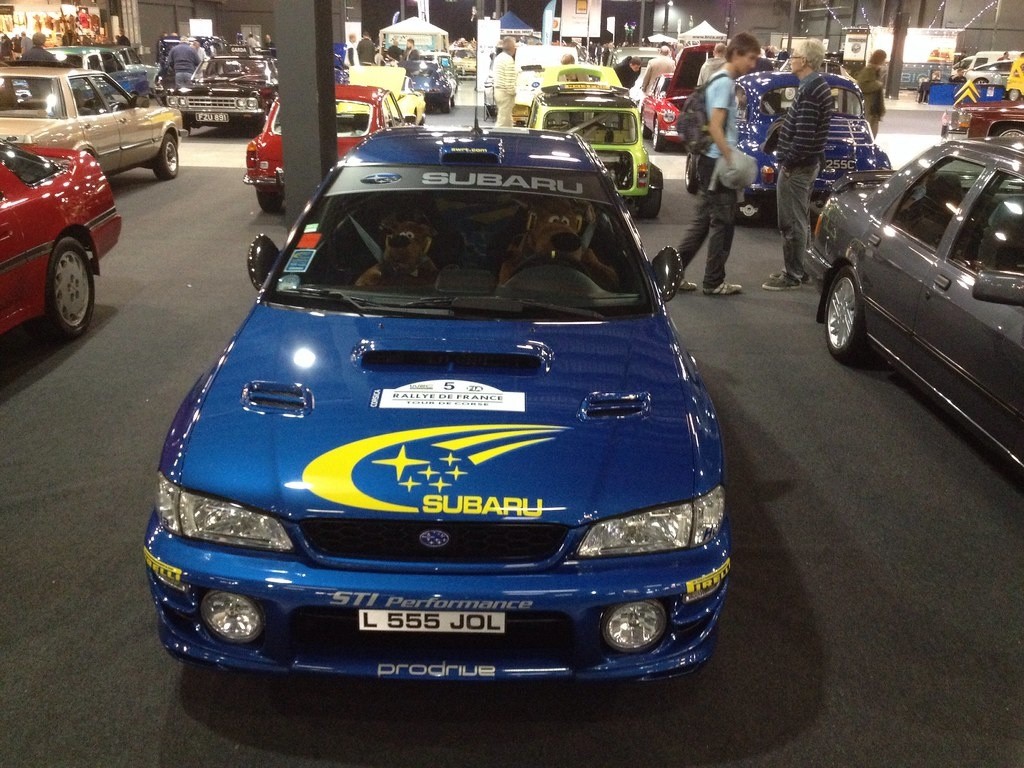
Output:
[770, 269, 809, 282]
[761, 275, 801, 290]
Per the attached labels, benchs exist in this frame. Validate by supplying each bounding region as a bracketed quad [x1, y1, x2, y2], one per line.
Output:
[906, 173, 1024, 260]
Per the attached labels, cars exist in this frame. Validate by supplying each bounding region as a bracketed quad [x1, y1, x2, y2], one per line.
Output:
[939, 101, 1024, 142]
[0, 36, 893, 217]
[964, 60, 1024, 87]
[0, 135, 125, 340]
[800, 135, 1024, 497]
[140, 124, 735, 694]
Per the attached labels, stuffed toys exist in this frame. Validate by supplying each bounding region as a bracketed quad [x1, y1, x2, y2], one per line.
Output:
[498, 197, 620, 293]
[354, 217, 458, 290]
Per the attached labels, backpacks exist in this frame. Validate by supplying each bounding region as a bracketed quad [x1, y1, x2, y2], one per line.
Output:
[676, 73, 729, 155]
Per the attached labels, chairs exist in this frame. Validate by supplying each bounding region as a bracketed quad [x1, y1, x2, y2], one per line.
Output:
[73, 88, 91, 115]
[977, 199, 1023, 268]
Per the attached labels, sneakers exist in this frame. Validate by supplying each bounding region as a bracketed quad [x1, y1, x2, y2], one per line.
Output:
[703, 282, 741, 294]
[678, 280, 698, 290]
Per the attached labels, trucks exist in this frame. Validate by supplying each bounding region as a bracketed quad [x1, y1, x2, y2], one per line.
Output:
[949, 50, 1024, 83]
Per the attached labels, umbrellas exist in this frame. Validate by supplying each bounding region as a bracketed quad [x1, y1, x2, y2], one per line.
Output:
[642, 34, 678, 44]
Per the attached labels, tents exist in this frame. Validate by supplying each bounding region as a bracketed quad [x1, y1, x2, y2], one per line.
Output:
[497, 10, 533, 37]
[378, 16, 449, 66]
[678, 20, 727, 46]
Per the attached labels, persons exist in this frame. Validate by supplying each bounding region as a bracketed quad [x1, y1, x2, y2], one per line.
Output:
[678, 31, 761, 296]
[856, 50, 888, 140]
[491, 37, 590, 128]
[0, 31, 59, 99]
[763, 37, 833, 290]
[949, 68, 967, 83]
[697, 42, 727, 88]
[589, 41, 616, 67]
[246, 34, 277, 57]
[765, 46, 790, 61]
[343, 32, 420, 77]
[156, 32, 208, 89]
[995, 52, 1012, 71]
[118, 30, 130, 46]
[614, 46, 676, 96]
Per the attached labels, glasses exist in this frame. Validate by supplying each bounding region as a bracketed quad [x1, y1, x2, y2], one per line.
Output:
[789, 55, 803, 59]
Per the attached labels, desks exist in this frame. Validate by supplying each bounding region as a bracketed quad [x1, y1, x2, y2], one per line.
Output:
[918, 83, 1006, 106]
